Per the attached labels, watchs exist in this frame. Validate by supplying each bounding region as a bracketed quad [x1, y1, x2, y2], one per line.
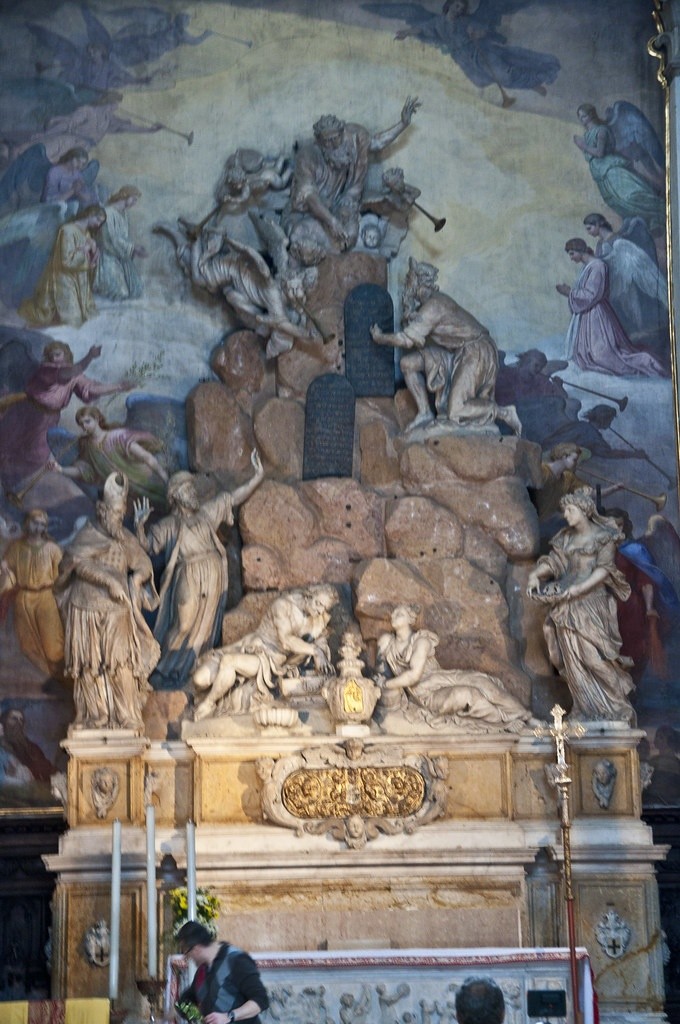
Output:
[228, 1011, 234, 1022]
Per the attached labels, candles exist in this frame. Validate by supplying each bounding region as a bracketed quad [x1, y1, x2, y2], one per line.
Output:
[145, 804, 157, 978]
[186, 818, 196, 921]
[109, 816, 121, 999]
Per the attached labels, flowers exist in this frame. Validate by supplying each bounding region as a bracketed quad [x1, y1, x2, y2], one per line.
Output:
[169, 885, 223, 940]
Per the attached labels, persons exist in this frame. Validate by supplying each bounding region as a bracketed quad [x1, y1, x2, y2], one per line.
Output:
[310, 980, 456, 1024]
[48, 96, 638, 733]
[165, 921, 270, 1024]
[455, 978, 505, 1024]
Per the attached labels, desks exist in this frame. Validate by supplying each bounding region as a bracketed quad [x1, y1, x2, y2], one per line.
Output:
[164, 946, 599, 1024]
